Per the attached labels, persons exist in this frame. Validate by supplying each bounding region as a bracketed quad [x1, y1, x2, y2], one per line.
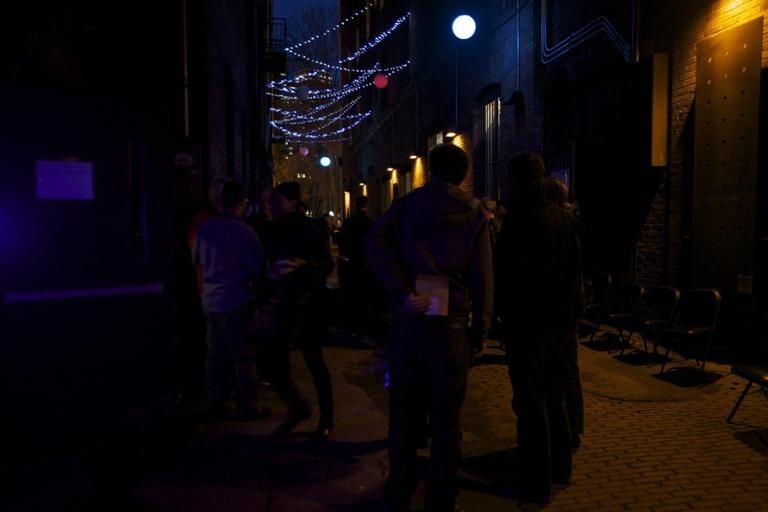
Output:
[187, 175, 335, 439]
[357, 145, 498, 512]
[459, 194, 507, 239]
[342, 192, 372, 328]
[544, 172, 586, 450]
[488, 150, 576, 501]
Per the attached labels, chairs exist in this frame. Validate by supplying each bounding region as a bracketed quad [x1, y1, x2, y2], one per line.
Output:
[574, 267, 723, 388]
[725, 327, 768, 424]
